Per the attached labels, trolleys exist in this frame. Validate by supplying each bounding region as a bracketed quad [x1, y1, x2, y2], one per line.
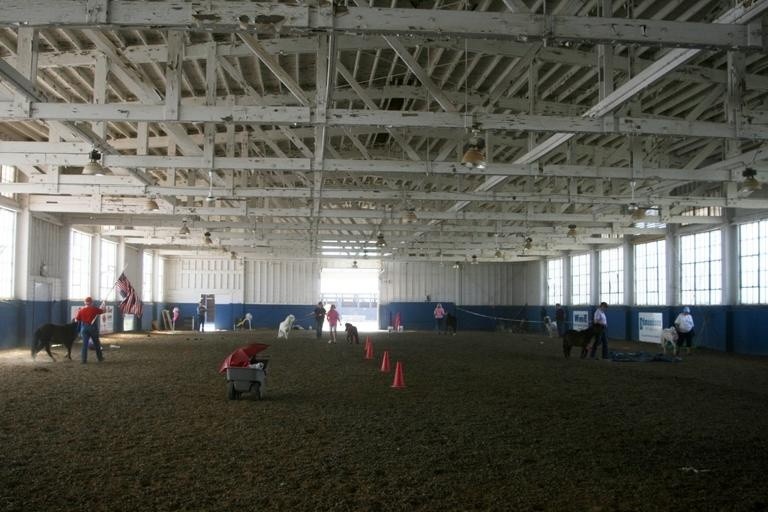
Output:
[223, 352, 272, 402]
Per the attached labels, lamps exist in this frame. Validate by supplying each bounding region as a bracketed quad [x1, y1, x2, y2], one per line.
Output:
[178, 220, 192, 234]
[375, 232, 386, 247]
[80, 143, 103, 175]
[460, 116, 487, 168]
[204, 233, 212, 245]
[568, 225, 576, 235]
[352, 260, 359, 268]
[741, 167, 758, 187]
[523, 237, 533, 251]
[628, 181, 639, 210]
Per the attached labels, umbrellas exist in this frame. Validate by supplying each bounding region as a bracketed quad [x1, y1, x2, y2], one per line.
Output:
[218, 343, 271, 374]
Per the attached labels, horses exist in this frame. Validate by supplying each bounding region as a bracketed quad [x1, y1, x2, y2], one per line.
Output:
[660, 326, 679, 356]
[31, 318, 82, 362]
[445, 311, 458, 335]
[563, 322, 606, 358]
[346, 323, 360, 345]
[277, 314, 296, 340]
[545, 317, 558, 336]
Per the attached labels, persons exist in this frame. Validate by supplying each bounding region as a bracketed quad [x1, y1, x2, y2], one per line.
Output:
[555, 303, 566, 338]
[315, 302, 326, 337]
[75, 297, 106, 364]
[447, 303, 459, 336]
[434, 303, 448, 335]
[197, 301, 206, 332]
[674, 306, 695, 356]
[590, 302, 611, 359]
[327, 305, 342, 344]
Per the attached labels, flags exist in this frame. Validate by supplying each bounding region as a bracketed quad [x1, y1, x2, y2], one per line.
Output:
[115, 273, 144, 319]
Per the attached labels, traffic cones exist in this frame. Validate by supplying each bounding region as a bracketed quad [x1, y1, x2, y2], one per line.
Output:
[363, 336, 375, 361]
[390, 358, 409, 390]
[377, 350, 390, 374]
